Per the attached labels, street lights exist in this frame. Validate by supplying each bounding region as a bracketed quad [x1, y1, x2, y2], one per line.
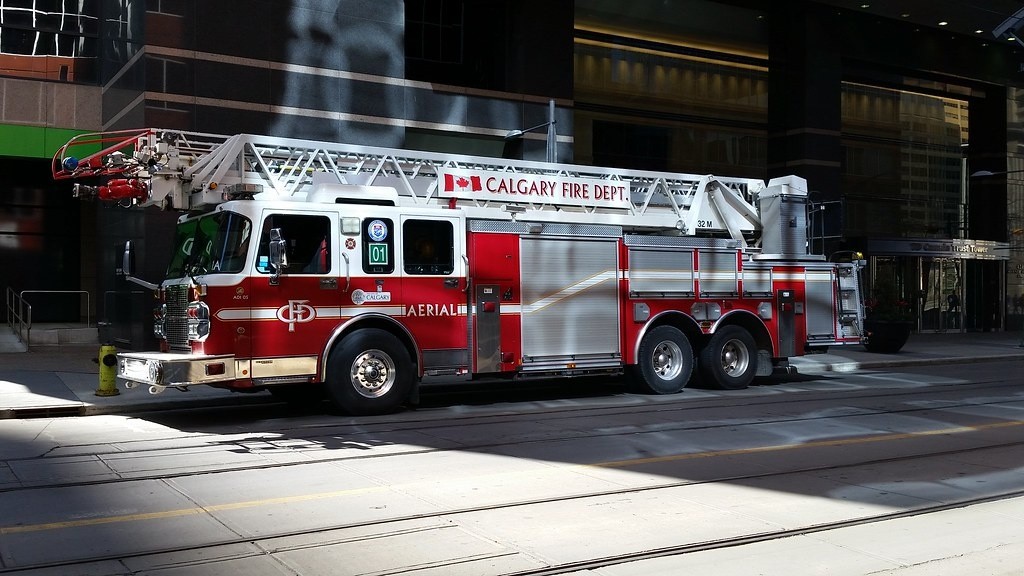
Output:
[504, 99, 555, 163]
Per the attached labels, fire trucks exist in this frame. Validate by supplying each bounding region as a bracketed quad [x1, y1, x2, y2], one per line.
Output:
[50, 127, 874, 417]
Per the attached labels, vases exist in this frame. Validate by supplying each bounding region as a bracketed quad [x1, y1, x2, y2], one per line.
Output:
[870, 321, 914, 355]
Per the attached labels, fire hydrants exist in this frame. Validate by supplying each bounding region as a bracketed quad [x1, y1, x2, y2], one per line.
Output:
[92, 342, 120, 397]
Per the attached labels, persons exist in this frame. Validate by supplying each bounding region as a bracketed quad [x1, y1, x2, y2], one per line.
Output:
[1013, 293, 1024, 318]
[948, 290, 961, 328]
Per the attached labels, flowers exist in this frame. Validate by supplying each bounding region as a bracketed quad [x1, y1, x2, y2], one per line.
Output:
[863, 297, 910, 320]
